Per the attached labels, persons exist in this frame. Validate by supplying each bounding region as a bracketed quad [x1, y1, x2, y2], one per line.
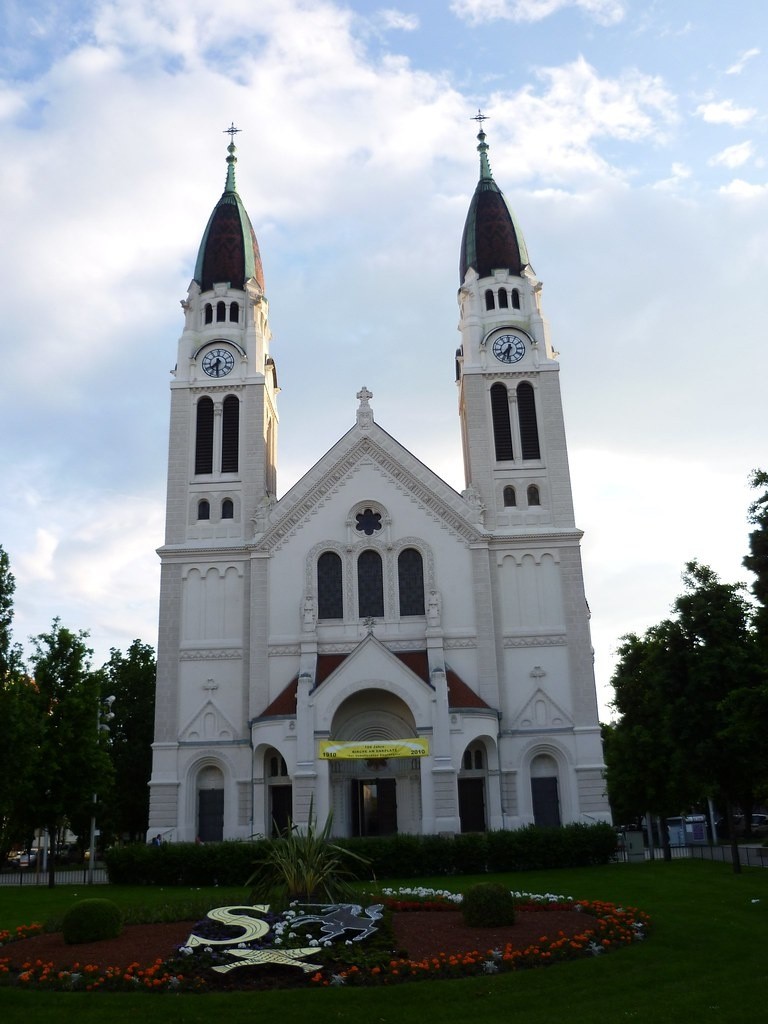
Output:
[152, 834, 162, 847]
[195, 835, 204, 845]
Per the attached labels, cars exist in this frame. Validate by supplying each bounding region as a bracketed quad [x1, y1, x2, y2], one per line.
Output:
[18, 849, 37, 868]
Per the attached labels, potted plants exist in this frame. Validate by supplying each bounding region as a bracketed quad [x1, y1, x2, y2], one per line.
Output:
[247, 791, 371, 905]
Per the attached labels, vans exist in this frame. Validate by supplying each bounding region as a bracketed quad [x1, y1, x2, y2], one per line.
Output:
[666, 815, 707, 848]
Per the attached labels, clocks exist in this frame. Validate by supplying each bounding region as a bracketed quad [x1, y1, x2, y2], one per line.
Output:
[202, 349, 234, 378]
[493, 334, 526, 364]
